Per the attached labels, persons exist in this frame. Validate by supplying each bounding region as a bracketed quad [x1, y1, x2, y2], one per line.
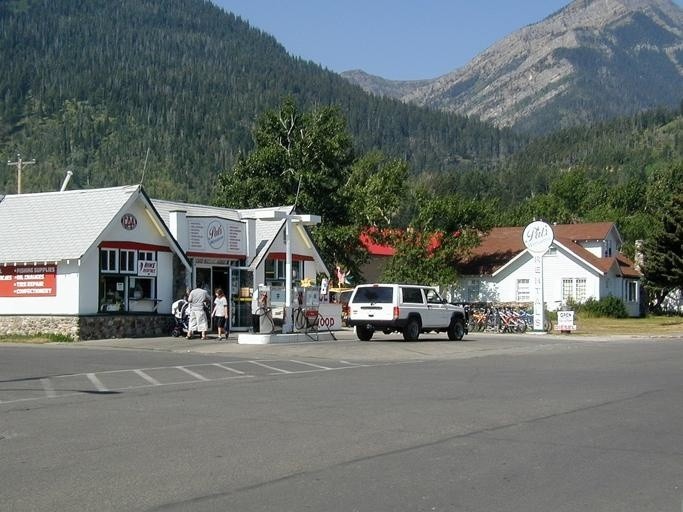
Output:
[251, 283, 264, 333]
[181, 282, 211, 340]
[211, 288, 229, 342]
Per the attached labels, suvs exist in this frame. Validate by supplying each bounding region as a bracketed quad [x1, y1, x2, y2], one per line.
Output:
[347, 284, 468, 341]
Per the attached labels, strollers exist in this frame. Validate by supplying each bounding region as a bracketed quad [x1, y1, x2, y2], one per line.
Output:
[172, 299, 190, 337]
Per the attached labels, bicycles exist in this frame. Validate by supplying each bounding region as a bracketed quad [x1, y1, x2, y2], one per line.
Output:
[468, 306, 552, 333]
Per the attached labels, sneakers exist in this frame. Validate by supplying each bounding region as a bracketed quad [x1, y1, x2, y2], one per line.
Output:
[226, 331, 229, 339]
[201, 336, 208, 340]
[187, 334, 191, 339]
[216, 336, 222, 341]
